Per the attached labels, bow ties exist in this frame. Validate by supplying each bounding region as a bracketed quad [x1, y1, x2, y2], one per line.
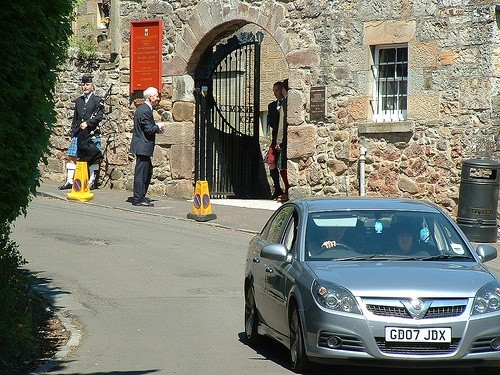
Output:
[278, 100, 282, 106]
[84, 95, 89, 99]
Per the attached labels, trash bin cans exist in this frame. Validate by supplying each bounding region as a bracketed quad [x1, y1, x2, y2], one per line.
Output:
[456, 159, 500, 242]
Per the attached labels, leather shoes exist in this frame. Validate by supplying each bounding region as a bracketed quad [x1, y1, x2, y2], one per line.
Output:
[89, 182, 99, 190]
[58, 182, 72, 190]
[132, 197, 155, 207]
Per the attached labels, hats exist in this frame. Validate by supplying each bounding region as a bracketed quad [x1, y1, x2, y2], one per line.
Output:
[82, 74, 92, 83]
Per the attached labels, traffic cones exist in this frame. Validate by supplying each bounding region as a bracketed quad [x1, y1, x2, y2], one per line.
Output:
[66, 162, 95, 202]
[187, 180, 217, 222]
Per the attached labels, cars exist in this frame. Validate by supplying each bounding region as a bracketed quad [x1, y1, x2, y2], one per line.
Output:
[243, 196, 500, 375]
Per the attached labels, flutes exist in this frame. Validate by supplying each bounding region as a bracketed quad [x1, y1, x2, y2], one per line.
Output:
[87, 83, 114, 135]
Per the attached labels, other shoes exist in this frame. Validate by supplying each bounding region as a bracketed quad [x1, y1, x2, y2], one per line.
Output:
[270, 189, 284, 200]
[277, 192, 289, 202]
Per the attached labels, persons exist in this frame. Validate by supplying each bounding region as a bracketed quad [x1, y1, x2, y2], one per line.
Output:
[59, 75, 104, 189]
[321, 227, 347, 250]
[393, 223, 439, 256]
[131, 87, 165, 207]
[266, 79, 289, 202]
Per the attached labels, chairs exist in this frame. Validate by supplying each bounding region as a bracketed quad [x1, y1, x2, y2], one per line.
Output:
[364, 217, 392, 246]
